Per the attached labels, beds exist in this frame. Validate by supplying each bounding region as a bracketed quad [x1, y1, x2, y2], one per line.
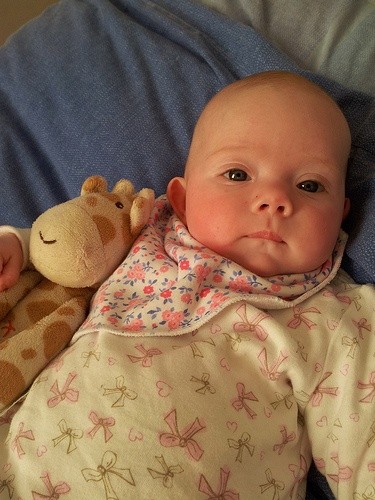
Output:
[0, 0, 375, 285]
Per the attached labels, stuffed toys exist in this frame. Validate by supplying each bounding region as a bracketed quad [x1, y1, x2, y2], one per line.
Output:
[0, 176, 155, 412]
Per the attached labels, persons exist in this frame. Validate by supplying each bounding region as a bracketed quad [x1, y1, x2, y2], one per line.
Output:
[0, 70, 375, 500]
